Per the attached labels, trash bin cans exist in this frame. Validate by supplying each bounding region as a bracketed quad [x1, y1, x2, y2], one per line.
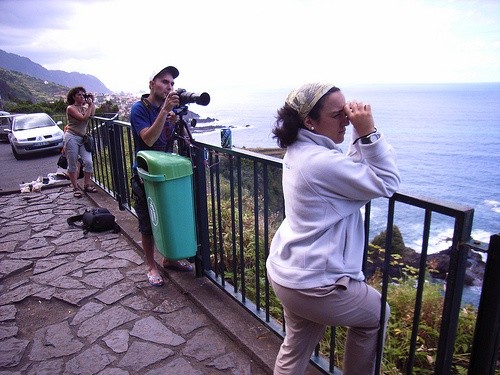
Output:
[136, 150, 198, 260]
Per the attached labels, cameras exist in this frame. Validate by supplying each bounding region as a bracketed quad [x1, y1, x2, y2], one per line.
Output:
[83, 94, 90, 98]
[173, 88, 210, 109]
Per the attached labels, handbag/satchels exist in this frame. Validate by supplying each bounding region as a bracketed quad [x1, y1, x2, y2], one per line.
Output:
[84, 136, 95, 152]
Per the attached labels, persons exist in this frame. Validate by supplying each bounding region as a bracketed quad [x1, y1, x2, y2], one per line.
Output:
[130, 66, 193, 286]
[64, 87, 98, 198]
[266, 82, 401, 375]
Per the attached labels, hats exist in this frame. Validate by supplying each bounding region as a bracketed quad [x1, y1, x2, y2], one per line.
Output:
[149, 65, 180, 81]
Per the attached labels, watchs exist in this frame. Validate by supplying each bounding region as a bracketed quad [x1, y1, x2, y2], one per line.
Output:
[361, 133, 381, 144]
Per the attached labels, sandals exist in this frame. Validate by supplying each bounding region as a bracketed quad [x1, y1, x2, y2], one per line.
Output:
[73, 188, 82, 197]
[84, 184, 98, 193]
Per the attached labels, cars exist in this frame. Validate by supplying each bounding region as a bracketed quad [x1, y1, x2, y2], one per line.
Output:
[0, 111, 15, 145]
[3, 113, 65, 159]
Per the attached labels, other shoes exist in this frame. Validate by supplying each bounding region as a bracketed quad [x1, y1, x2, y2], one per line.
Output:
[146, 265, 164, 286]
[163, 258, 194, 272]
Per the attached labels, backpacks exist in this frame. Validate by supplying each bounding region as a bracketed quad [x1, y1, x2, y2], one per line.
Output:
[66, 208, 120, 235]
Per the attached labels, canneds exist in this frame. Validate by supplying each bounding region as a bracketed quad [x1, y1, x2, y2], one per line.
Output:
[221, 128, 231, 148]
